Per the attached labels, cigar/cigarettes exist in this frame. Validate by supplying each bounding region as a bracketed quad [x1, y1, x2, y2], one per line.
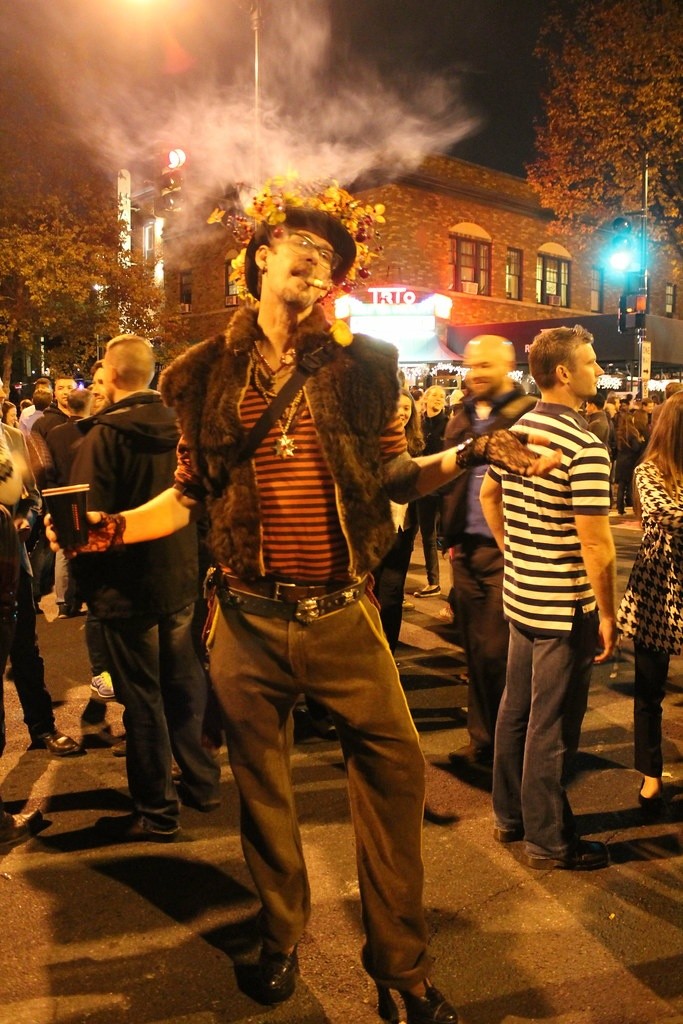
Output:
[299, 274, 333, 290]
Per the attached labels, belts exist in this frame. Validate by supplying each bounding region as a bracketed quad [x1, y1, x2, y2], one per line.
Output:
[215, 572, 372, 623]
[211, 570, 353, 600]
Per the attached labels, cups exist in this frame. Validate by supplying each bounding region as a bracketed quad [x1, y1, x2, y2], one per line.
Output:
[41, 484, 92, 548]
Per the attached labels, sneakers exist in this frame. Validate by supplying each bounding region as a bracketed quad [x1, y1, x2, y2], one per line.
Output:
[58, 603, 71, 619]
[90, 670, 116, 698]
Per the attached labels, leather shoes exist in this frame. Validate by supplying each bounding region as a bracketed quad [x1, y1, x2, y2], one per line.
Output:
[32, 727, 78, 755]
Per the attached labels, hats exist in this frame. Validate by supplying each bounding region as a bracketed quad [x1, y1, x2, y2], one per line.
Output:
[243, 214, 356, 302]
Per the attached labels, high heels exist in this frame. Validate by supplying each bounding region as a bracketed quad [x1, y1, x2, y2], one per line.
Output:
[375, 977, 458, 1024]
[255, 937, 299, 1004]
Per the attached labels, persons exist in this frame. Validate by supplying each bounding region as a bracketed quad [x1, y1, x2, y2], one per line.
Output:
[42, 206, 563, 1024]
[479, 326, 618, 870]
[0, 336, 225, 840]
[615, 390, 683, 810]
[308, 370, 471, 738]
[436, 333, 536, 770]
[579, 382, 683, 515]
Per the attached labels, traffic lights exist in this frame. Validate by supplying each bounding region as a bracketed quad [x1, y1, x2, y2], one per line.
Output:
[616, 292, 650, 334]
[155, 148, 189, 215]
[609, 210, 635, 277]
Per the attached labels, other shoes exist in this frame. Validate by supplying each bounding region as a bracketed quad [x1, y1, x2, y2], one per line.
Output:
[493, 816, 585, 842]
[172, 766, 222, 811]
[413, 586, 441, 597]
[111, 740, 127, 755]
[527, 840, 610, 869]
[638, 774, 664, 809]
[447, 752, 493, 791]
[0, 807, 42, 846]
[460, 672, 470, 681]
[402, 599, 414, 611]
[96, 809, 177, 843]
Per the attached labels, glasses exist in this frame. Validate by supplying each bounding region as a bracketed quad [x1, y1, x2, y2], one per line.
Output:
[285, 229, 343, 272]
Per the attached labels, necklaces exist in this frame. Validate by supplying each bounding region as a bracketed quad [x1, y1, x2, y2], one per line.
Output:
[255, 344, 303, 459]
